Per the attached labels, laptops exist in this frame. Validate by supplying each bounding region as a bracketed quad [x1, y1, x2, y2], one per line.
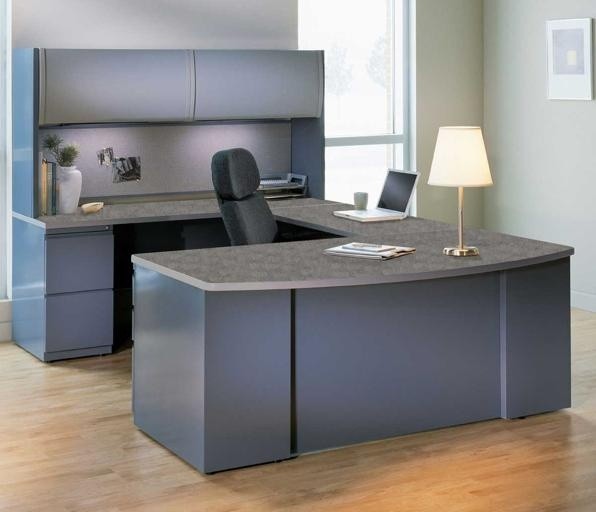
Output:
[334, 168, 421, 223]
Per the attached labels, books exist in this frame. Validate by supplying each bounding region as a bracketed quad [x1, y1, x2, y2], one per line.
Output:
[38, 153, 57, 217]
[322, 241, 417, 261]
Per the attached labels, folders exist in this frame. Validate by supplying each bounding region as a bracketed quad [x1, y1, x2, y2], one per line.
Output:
[40, 159, 57, 215]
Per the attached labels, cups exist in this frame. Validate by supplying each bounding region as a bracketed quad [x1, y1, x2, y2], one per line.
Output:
[353, 190, 369, 210]
